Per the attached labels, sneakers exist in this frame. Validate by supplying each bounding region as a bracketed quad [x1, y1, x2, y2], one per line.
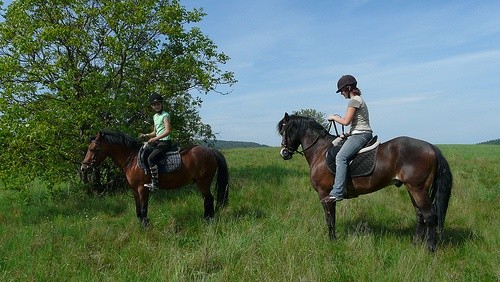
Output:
[321, 192, 343, 202]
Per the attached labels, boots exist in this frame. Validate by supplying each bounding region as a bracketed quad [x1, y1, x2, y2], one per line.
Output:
[144, 165, 159, 191]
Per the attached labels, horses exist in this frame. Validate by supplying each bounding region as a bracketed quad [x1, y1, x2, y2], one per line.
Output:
[80, 130, 230, 228]
[275, 111, 454, 254]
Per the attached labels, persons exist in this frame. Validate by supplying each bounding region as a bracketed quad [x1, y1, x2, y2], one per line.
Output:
[320, 75, 373, 204]
[139, 93, 171, 190]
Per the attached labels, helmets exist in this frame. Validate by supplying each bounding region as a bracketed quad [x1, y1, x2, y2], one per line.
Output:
[336, 75, 357, 93]
[147, 92, 163, 103]
